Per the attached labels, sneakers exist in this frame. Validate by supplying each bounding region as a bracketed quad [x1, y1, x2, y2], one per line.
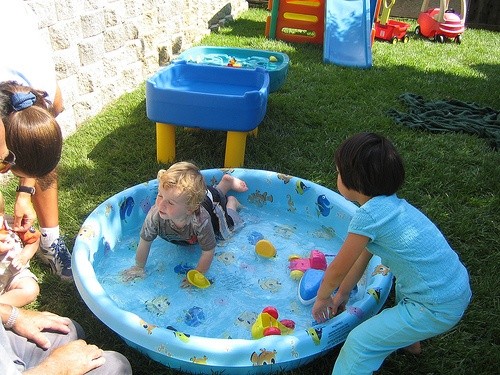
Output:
[36, 238, 74, 283]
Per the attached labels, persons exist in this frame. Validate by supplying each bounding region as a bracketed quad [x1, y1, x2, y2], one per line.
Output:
[0, 0, 133, 375]
[119, 161, 249, 288]
[311, 133, 473, 375]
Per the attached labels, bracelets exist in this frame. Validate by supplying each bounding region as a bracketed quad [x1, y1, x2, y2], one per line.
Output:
[3, 305, 19, 330]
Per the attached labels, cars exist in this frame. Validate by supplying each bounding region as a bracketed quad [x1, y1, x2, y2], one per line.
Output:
[414, 7, 465, 45]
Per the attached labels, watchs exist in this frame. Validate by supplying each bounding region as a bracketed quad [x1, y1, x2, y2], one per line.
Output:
[16, 185, 36, 195]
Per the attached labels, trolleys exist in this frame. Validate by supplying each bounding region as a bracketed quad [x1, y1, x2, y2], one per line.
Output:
[374, 0, 411, 44]
